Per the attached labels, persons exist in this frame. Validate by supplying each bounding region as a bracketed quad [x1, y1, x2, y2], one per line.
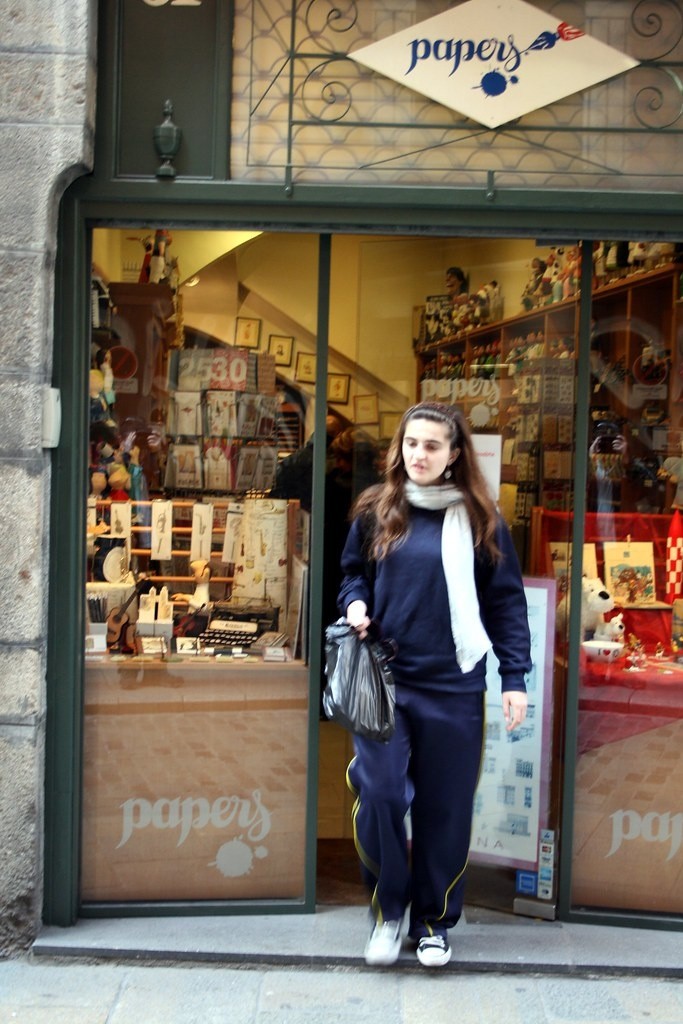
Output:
[267, 412, 391, 683]
[171, 560, 212, 630]
[118, 418, 179, 500]
[339, 400, 533, 968]
[585, 406, 661, 514]
[138, 226, 179, 294]
[418, 240, 581, 397]
[86, 343, 132, 505]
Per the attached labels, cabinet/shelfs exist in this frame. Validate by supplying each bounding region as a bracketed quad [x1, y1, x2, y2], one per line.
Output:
[108, 281, 176, 500]
[415, 264, 683, 577]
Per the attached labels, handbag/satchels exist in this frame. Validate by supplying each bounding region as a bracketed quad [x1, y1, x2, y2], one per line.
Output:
[323, 617, 397, 739]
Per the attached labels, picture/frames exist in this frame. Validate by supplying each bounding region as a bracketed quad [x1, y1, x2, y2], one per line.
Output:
[326, 372, 351, 405]
[379, 412, 406, 439]
[234, 316, 262, 349]
[268, 334, 295, 367]
[353, 392, 381, 426]
[294, 351, 316, 386]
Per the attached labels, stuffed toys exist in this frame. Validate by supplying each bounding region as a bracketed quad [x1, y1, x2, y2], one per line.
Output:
[592, 612, 625, 643]
[557, 575, 613, 644]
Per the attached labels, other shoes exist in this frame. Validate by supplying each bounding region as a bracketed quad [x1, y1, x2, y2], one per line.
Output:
[417, 937, 451, 966]
[365, 923, 401, 965]
